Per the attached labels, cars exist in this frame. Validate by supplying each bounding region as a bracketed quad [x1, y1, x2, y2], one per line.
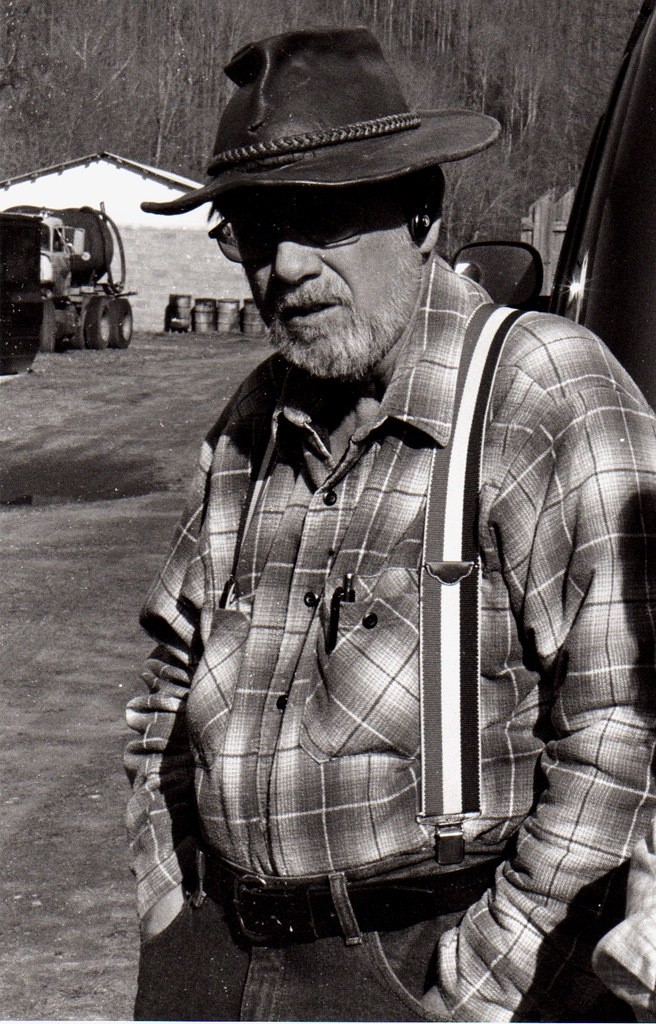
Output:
[456, 5, 656, 416]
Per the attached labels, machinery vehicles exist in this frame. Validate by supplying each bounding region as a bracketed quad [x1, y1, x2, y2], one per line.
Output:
[0, 202, 134, 352]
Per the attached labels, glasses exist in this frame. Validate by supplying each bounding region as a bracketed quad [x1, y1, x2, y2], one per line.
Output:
[209, 184, 399, 263]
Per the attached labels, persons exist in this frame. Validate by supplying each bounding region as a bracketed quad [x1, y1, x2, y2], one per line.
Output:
[121, 22, 656, 1024]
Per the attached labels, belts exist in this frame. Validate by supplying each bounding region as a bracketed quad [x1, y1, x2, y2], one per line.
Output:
[204, 856, 510, 944]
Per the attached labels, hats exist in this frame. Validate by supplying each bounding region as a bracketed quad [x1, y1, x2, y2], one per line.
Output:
[141, 27, 503, 215]
